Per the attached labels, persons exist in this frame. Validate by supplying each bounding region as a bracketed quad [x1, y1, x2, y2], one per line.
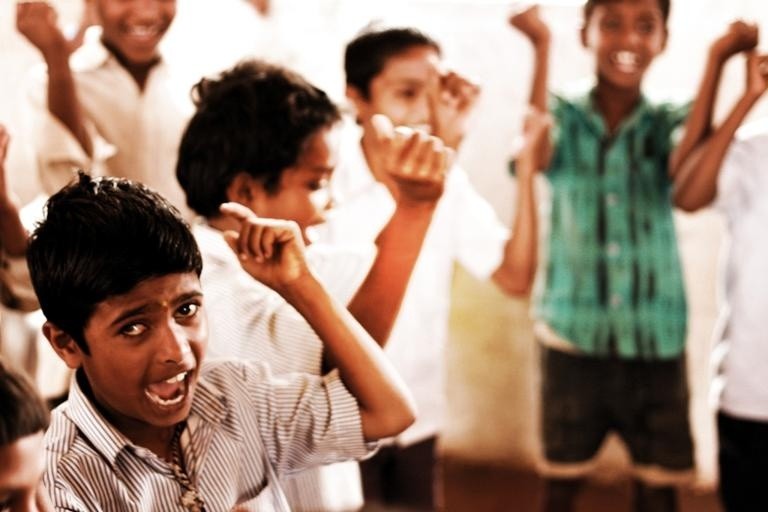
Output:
[165, 60, 447, 387]
[305, 27, 551, 512]
[667, 49, 767, 510]
[16, 0, 194, 222]
[1, 358, 57, 512]
[26, 178, 419, 511]
[496, 1, 767, 512]
